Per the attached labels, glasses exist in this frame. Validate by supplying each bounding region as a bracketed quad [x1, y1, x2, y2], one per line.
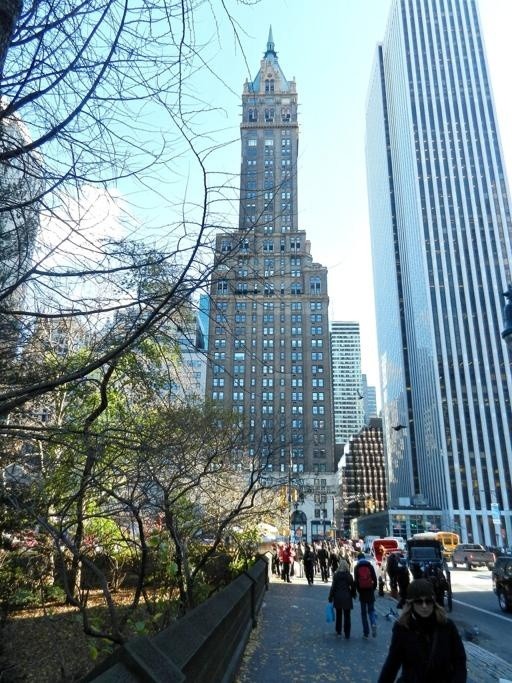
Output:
[410, 597, 435, 605]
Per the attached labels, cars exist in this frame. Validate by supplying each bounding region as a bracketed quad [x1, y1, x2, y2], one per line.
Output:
[359, 530, 512, 616]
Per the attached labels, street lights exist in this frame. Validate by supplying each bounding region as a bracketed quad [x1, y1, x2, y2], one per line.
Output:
[499, 280, 511, 378]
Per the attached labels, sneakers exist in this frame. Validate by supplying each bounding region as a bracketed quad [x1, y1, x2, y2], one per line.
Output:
[363, 624, 377, 640]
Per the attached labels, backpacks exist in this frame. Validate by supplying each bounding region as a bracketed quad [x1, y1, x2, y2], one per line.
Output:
[358, 566, 374, 588]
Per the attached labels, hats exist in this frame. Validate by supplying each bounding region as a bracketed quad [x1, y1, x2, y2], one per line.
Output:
[336, 559, 349, 572]
[404, 579, 435, 600]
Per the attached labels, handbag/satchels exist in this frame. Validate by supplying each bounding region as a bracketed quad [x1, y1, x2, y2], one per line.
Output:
[325, 602, 335, 623]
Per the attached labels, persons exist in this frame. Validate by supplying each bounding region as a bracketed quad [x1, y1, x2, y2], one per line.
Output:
[271, 537, 411, 640]
[376, 578, 467, 682]
